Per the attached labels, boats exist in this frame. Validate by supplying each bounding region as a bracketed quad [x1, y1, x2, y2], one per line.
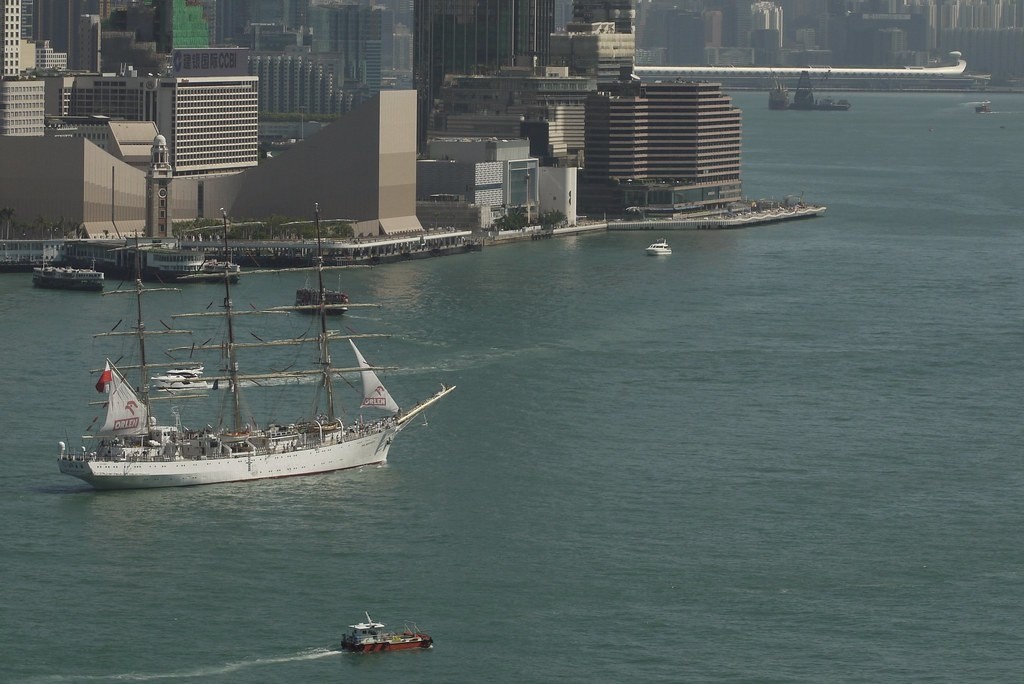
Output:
[341, 611, 435, 651]
[166, 365, 205, 378]
[150, 373, 208, 392]
[30, 264, 106, 291]
[646, 238, 673, 256]
[788, 70, 850, 111]
[294, 287, 350, 316]
[768, 77, 790, 111]
[975, 104, 992, 114]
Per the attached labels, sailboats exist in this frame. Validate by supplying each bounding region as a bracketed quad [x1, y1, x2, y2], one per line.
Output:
[58, 201, 459, 493]
